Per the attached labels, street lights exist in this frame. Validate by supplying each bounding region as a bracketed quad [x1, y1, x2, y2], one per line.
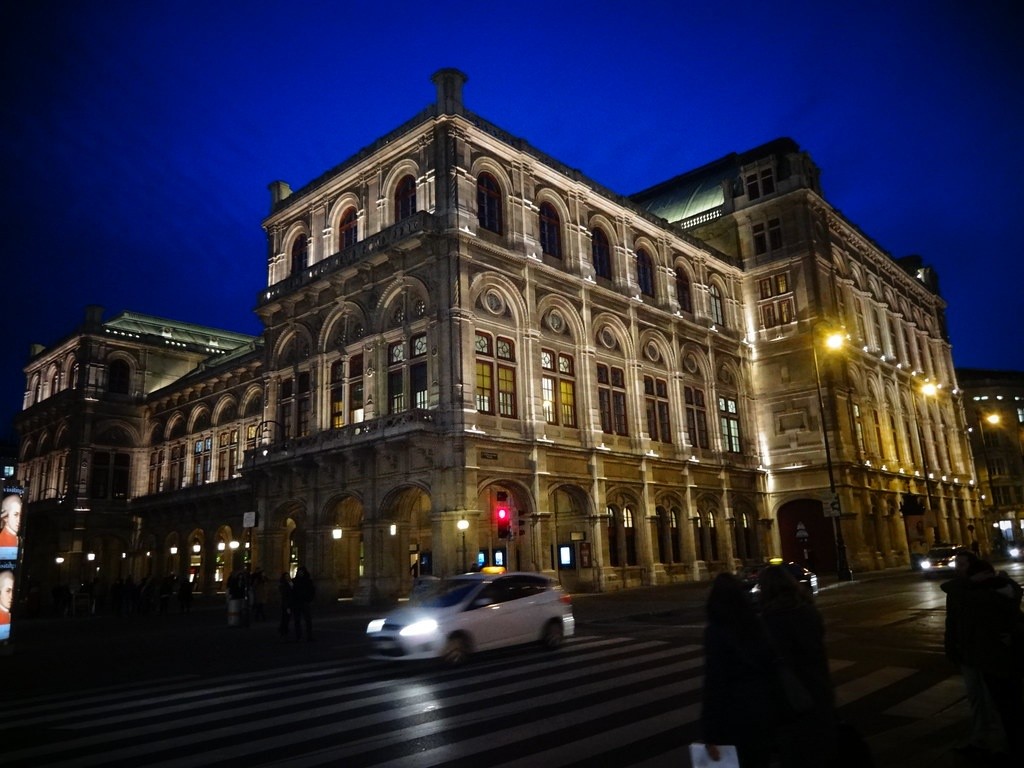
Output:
[978, 406, 1008, 557]
[795, 521, 813, 574]
[243, 421, 290, 627]
[909, 369, 943, 544]
[809, 318, 855, 581]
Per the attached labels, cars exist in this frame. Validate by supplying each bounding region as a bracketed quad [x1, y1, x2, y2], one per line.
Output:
[733, 562, 820, 599]
[920, 544, 966, 578]
[1011, 539, 1024, 561]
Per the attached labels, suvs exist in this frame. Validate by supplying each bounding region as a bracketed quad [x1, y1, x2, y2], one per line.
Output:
[362, 573, 575, 669]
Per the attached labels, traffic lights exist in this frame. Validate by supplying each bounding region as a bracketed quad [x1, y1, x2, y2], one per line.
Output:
[514, 510, 525, 538]
[496, 508, 509, 538]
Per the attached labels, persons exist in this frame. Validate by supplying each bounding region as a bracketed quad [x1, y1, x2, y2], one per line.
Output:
[278, 572, 295, 643]
[699, 572, 794, 768]
[993, 536, 1009, 556]
[943, 550, 1024, 768]
[25, 562, 264, 625]
[972, 539, 981, 558]
[291, 565, 316, 642]
[408, 560, 418, 580]
[756, 574, 833, 768]
[470, 562, 487, 573]
